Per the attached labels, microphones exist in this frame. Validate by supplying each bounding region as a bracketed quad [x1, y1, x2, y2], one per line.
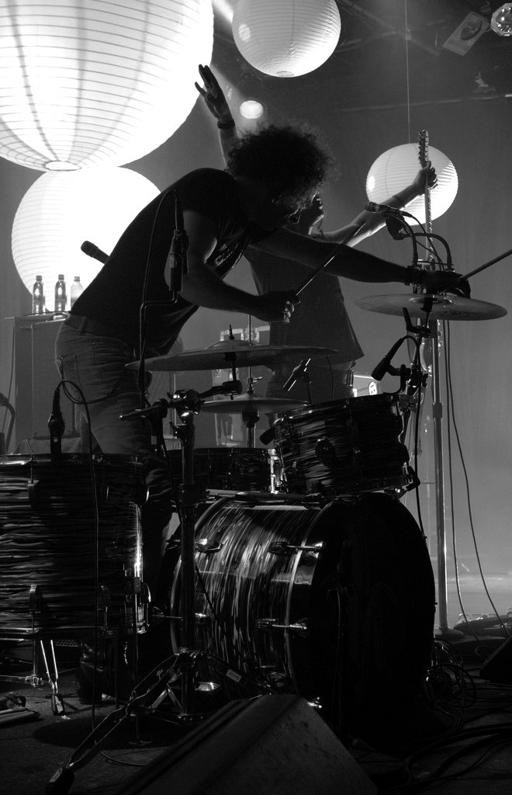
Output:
[48, 388, 64, 436]
[365, 202, 411, 216]
[282, 355, 311, 393]
[372, 338, 403, 381]
[175, 194, 190, 275]
[82, 241, 109, 264]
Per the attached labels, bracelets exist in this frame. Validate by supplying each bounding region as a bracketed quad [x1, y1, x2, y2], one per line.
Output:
[217, 119, 236, 129]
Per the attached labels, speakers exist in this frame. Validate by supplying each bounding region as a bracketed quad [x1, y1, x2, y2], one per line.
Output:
[112, 694, 378, 795]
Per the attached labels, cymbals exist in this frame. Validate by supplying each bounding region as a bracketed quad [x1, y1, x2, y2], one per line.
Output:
[356, 294, 507, 320]
[124, 341, 343, 371]
[198, 394, 309, 414]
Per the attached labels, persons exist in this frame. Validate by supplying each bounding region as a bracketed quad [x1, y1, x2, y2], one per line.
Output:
[194, 65, 366, 399]
[55, 121, 471, 454]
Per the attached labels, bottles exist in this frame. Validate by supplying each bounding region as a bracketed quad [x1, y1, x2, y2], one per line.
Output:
[55, 273, 84, 311]
[33, 274, 44, 313]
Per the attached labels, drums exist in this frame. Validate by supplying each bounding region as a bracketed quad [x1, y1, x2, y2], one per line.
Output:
[140, 451, 276, 615]
[164, 490, 435, 700]
[268, 393, 410, 497]
[2, 449, 155, 644]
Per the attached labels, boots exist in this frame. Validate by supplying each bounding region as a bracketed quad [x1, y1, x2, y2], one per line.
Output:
[77, 556, 129, 702]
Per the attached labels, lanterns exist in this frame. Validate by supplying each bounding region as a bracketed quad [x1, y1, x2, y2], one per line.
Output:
[11, 170, 161, 310]
[0, 0, 215, 169]
[231, 0, 341, 78]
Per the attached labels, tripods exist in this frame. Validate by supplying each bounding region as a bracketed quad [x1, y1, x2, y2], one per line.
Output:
[45, 362, 280, 795]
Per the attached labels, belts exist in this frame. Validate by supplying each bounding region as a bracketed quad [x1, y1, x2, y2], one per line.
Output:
[322, 371, 353, 386]
[65, 313, 131, 341]
